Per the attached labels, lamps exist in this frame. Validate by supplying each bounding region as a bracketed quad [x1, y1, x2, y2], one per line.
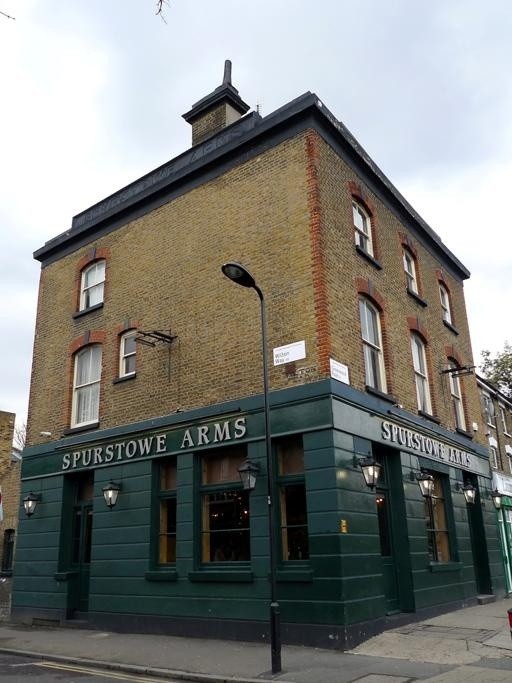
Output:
[102, 478, 122, 511]
[456, 477, 478, 508]
[23, 491, 42, 518]
[486, 486, 502, 513]
[409, 469, 435, 501]
[352, 450, 384, 491]
[237, 454, 261, 493]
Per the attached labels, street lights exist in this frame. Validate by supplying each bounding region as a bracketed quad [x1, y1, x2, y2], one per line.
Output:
[215, 260, 290, 673]
